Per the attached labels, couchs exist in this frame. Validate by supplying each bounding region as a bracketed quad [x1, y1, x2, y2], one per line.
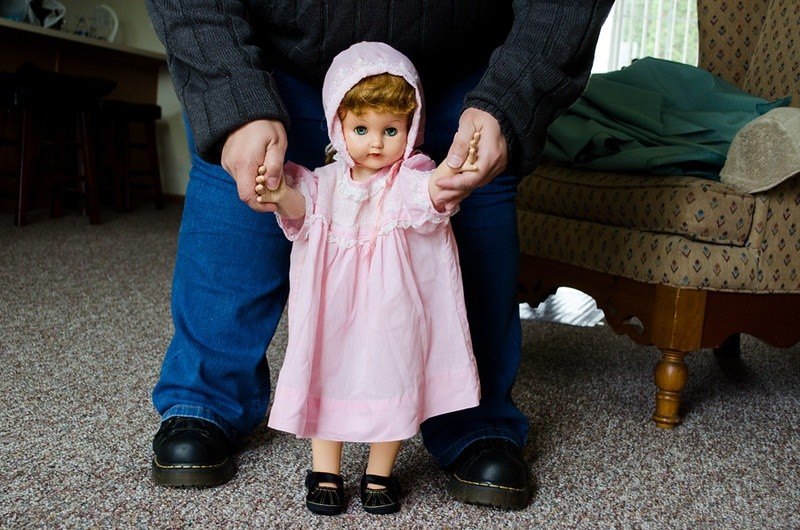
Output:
[516, 0, 800, 427]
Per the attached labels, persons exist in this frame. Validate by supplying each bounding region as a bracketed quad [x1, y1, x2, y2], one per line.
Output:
[146, 0, 615, 511]
[254, 41, 480, 515]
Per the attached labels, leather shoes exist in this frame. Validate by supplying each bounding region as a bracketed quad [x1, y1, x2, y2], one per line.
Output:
[151, 418, 238, 488]
[448, 439, 537, 510]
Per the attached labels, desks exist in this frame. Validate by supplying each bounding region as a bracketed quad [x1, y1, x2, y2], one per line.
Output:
[0, 18, 168, 212]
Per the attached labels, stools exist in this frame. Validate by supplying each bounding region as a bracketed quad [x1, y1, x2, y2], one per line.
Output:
[0, 60, 117, 227]
[51, 99, 165, 218]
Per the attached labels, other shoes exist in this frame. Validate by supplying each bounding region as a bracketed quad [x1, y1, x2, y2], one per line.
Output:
[303, 469, 344, 515]
[360, 468, 401, 514]
